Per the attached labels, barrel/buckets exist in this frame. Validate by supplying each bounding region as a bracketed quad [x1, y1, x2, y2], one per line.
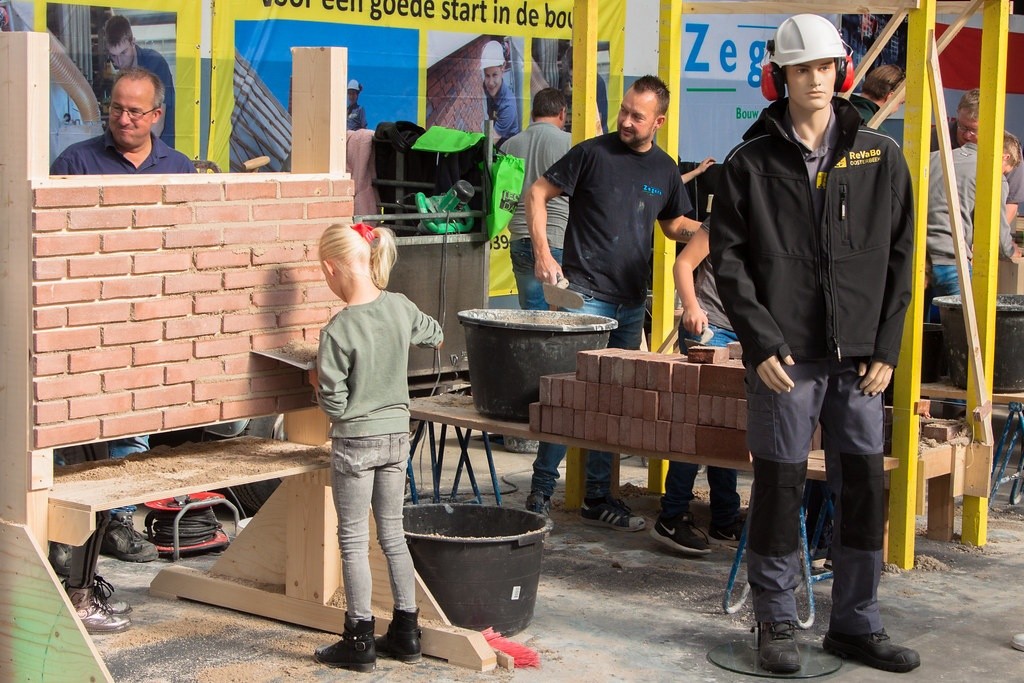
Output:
[400, 501, 554, 639]
[932, 295, 1024, 394]
[457, 309, 619, 423]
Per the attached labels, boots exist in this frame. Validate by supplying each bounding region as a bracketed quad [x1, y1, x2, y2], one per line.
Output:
[92, 572, 131, 615]
[314, 612, 376, 673]
[61, 580, 132, 634]
[376, 605, 423, 663]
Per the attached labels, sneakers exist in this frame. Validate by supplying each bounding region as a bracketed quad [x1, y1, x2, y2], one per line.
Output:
[526, 491, 551, 517]
[757, 620, 800, 672]
[650, 512, 711, 554]
[823, 629, 921, 671]
[708, 521, 746, 546]
[99, 512, 159, 562]
[578, 495, 645, 531]
[48, 542, 100, 577]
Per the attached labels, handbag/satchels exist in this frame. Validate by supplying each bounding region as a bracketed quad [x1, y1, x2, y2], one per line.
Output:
[482, 143, 525, 241]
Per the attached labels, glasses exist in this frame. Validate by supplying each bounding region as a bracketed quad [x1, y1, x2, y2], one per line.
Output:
[108, 104, 161, 120]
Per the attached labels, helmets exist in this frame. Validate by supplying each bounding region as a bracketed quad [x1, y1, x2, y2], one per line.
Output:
[770, 14, 847, 67]
[480, 41, 504, 69]
[347, 80, 360, 90]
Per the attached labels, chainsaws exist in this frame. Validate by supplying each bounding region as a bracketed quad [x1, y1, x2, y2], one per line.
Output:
[414, 179, 475, 234]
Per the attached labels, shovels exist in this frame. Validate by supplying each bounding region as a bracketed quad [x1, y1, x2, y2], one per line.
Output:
[684, 327, 714, 348]
[542, 276, 584, 310]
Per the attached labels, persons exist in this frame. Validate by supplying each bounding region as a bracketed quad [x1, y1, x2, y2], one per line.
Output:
[852, 14, 883, 78]
[347, 79, 368, 130]
[709, 12, 921, 675]
[104, 16, 175, 149]
[525, 75, 752, 555]
[498, 87, 572, 311]
[564, 48, 608, 140]
[308, 221, 445, 670]
[483, 40, 519, 151]
[927, 90, 1024, 323]
[50, 67, 197, 631]
[851, 65, 909, 133]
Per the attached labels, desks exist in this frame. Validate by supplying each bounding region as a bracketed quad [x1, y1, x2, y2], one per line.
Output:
[407, 394, 901, 629]
[912, 382, 1024, 507]
